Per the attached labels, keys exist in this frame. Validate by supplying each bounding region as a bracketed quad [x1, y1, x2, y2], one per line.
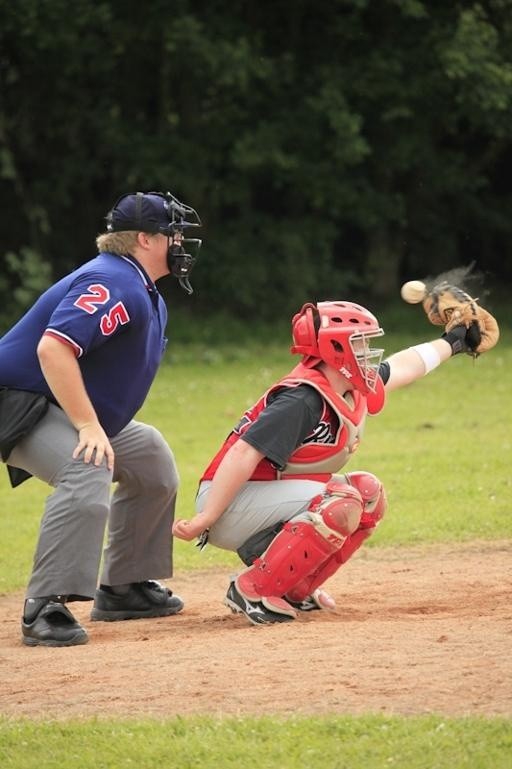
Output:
[193, 526, 210, 552]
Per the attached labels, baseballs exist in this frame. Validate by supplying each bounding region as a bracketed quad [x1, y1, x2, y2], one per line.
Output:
[401, 280, 427, 305]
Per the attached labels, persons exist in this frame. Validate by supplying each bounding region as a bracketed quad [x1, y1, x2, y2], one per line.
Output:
[0, 191, 203, 646]
[171, 302, 481, 626]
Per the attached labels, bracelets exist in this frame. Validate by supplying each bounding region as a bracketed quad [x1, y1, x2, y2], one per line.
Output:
[411, 343, 441, 376]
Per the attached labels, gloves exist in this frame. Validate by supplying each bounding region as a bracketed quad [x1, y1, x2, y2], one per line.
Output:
[441, 326, 471, 358]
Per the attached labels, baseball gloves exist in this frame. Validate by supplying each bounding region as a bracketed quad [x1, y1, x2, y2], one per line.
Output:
[423, 282, 500, 357]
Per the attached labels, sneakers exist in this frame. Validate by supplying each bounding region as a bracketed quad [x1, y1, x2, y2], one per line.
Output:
[21, 598, 88, 646]
[223, 581, 319, 624]
[90, 581, 183, 621]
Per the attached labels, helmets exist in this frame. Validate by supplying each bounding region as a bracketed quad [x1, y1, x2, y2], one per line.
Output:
[291, 301, 385, 396]
[104, 192, 202, 294]
